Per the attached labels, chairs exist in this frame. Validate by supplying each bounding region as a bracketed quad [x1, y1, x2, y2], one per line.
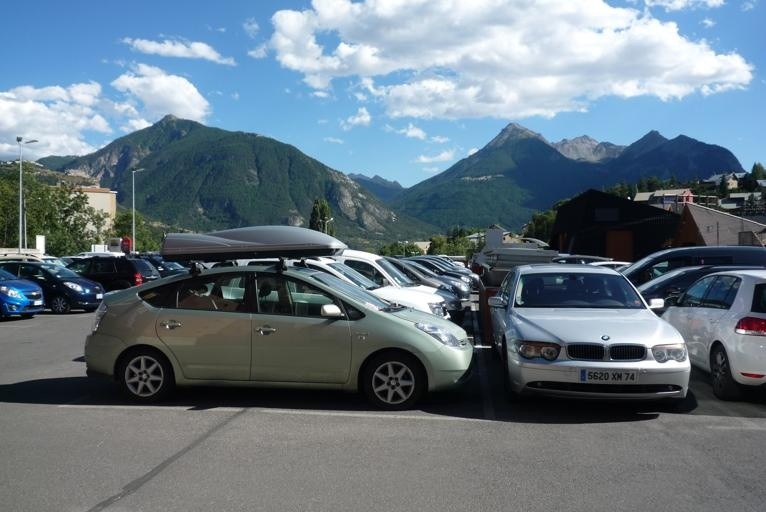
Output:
[260, 282, 278, 313]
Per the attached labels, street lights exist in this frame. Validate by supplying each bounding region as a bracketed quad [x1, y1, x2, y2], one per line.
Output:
[16, 136, 39, 254]
[325, 217, 333, 235]
[132, 168, 145, 253]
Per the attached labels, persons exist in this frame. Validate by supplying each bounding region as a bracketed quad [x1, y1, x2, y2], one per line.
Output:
[119, 231, 131, 255]
